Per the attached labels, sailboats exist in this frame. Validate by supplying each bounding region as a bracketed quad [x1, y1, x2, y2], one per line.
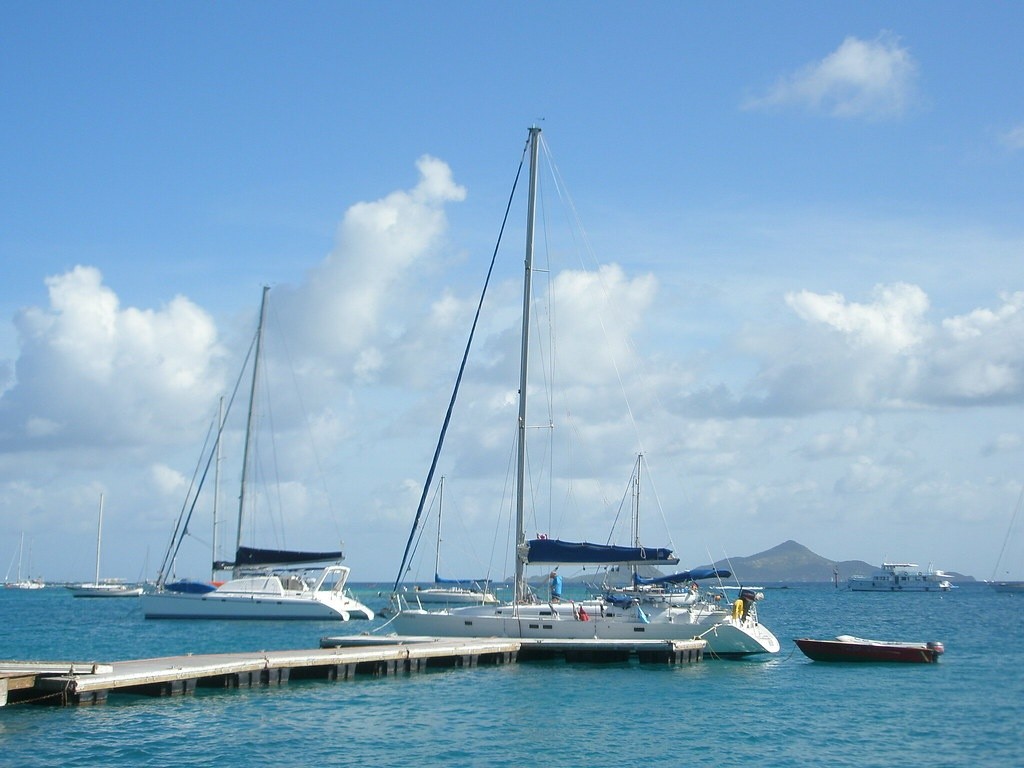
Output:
[377, 472, 501, 607]
[2, 532, 47, 591]
[582, 447, 734, 608]
[140, 287, 378, 623]
[371, 115, 784, 661]
[70, 490, 145, 597]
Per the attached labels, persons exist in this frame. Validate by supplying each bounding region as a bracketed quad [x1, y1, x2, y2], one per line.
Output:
[548, 571, 563, 620]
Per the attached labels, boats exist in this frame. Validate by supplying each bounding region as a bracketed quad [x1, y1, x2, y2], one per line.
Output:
[848, 561, 961, 592]
[792, 634, 945, 664]
[708, 584, 765, 590]
